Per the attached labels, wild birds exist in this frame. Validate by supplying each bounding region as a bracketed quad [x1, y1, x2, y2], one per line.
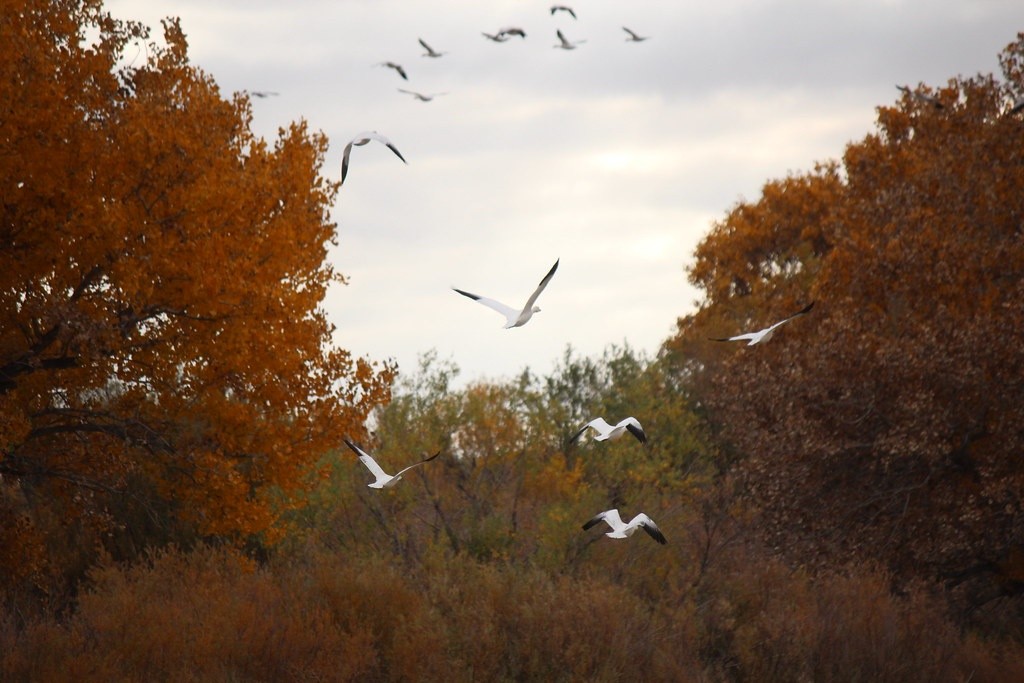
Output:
[622, 26, 651, 43]
[568, 417, 647, 446]
[581, 508, 670, 547]
[549, 5, 589, 50]
[381, 37, 450, 101]
[708, 301, 814, 348]
[482, 26, 528, 43]
[451, 257, 560, 330]
[251, 89, 282, 98]
[341, 435, 441, 490]
[341, 131, 409, 186]
